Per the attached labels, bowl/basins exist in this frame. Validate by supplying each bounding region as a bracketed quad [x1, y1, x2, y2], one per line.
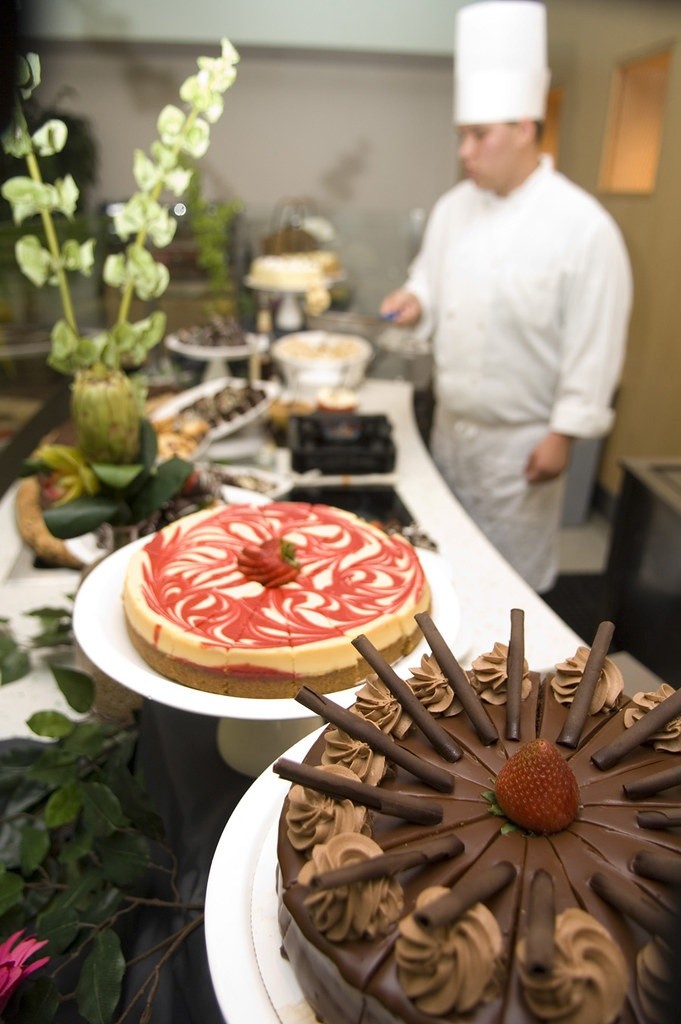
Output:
[272, 331, 374, 396]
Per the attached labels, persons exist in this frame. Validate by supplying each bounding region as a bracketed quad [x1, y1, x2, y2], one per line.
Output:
[379, 1, 632, 587]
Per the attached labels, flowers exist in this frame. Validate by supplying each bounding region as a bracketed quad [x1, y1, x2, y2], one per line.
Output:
[5, 34, 240, 375]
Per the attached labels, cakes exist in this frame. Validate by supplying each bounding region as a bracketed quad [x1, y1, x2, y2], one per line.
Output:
[247, 249, 343, 289]
[122, 500, 435, 700]
[270, 606, 681, 1024]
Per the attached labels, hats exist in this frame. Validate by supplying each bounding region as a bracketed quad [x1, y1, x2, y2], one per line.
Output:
[453, 2, 552, 126]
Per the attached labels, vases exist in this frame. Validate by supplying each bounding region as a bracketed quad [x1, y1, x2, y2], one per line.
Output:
[70, 366, 140, 468]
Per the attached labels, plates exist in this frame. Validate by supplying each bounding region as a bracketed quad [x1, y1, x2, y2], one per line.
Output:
[207, 461, 293, 502]
[150, 376, 278, 444]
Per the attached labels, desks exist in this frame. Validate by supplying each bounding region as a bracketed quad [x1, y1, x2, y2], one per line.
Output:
[0, 368, 591, 734]
